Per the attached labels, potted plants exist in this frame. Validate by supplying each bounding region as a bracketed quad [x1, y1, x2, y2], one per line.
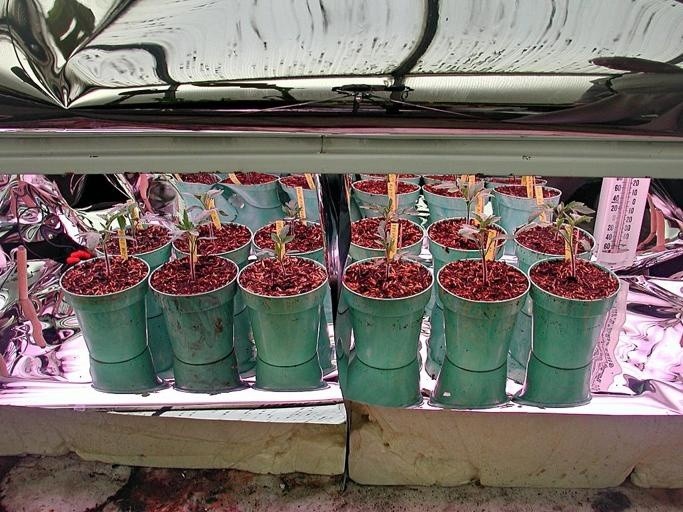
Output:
[252, 221, 323, 265]
[94, 202, 174, 319]
[171, 190, 252, 314]
[346, 217, 423, 261]
[217, 172, 279, 209]
[520, 214, 621, 404]
[177, 172, 218, 192]
[238, 225, 329, 391]
[426, 181, 487, 223]
[512, 222, 596, 317]
[149, 210, 243, 392]
[353, 180, 421, 226]
[489, 177, 548, 190]
[494, 186, 561, 256]
[59, 212, 167, 393]
[361, 173, 420, 186]
[278, 174, 321, 221]
[340, 229, 434, 407]
[428, 217, 508, 309]
[428, 211, 530, 407]
[423, 175, 481, 187]
